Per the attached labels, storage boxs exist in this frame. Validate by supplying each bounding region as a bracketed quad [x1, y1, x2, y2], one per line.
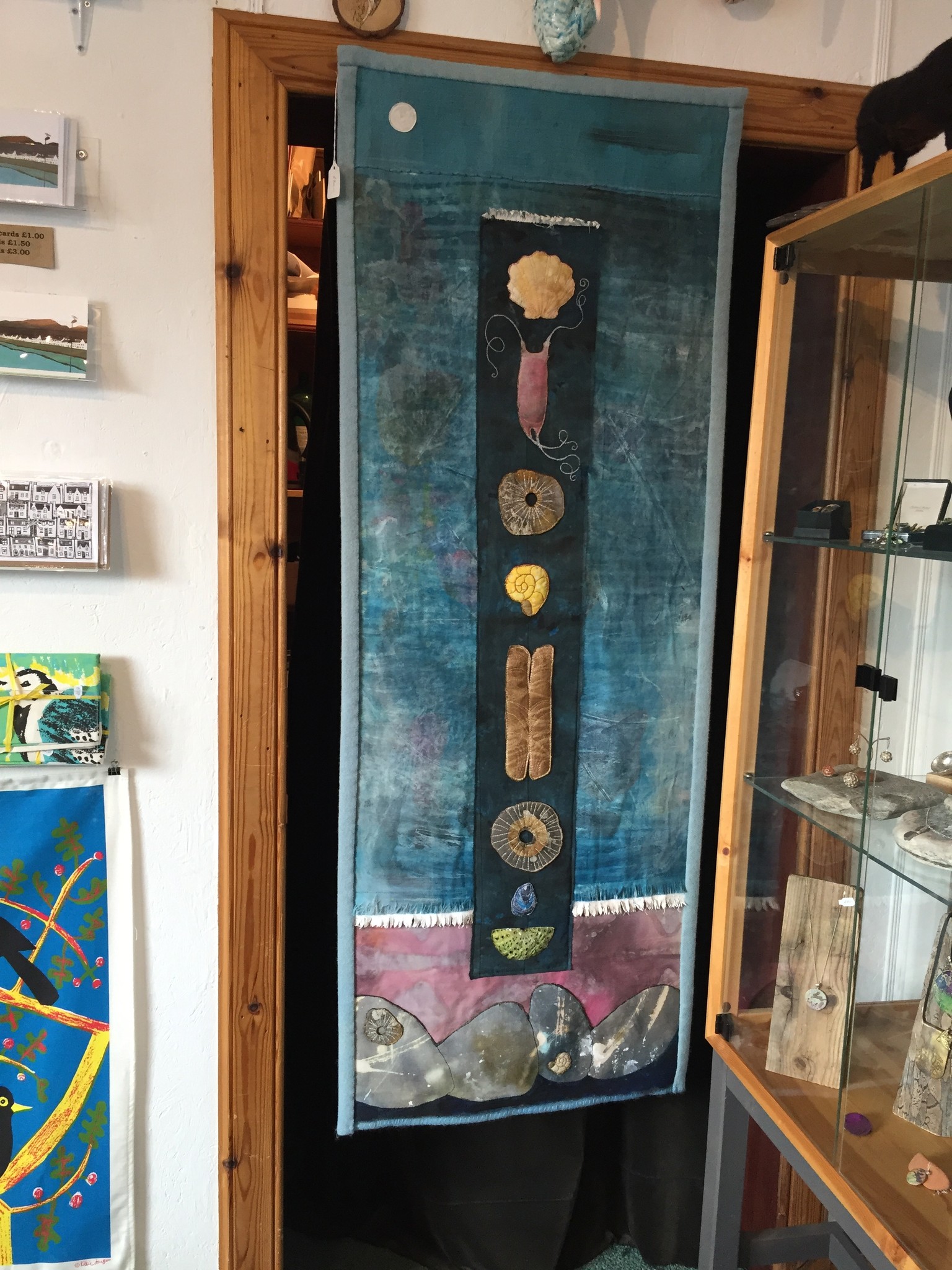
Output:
[794, 498, 852, 540]
[311, 178, 326, 219]
[288, 308, 318, 326]
[861, 478, 952, 542]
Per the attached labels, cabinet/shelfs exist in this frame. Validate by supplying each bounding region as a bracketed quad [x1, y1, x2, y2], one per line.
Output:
[286, 216, 325, 500]
[698, 160, 952, 1270]
